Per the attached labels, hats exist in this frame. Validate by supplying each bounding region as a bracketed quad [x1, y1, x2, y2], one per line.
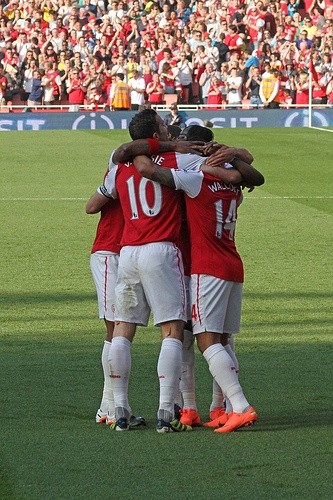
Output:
[113, 73, 124, 80]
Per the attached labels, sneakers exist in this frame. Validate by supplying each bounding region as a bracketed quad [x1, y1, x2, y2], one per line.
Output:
[95, 408, 108, 424]
[179, 408, 200, 425]
[214, 406, 258, 433]
[203, 407, 234, 428]
[115, 418, 130, 431]
[156, 418, 193, 433]
[105, 412, 145, 425]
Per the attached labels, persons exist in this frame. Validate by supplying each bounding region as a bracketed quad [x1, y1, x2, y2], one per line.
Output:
[162, 104, 183, 128]
[0, 0, 333, 433]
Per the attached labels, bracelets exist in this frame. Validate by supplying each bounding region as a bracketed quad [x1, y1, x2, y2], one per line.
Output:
[228, 156, 236, 164]
